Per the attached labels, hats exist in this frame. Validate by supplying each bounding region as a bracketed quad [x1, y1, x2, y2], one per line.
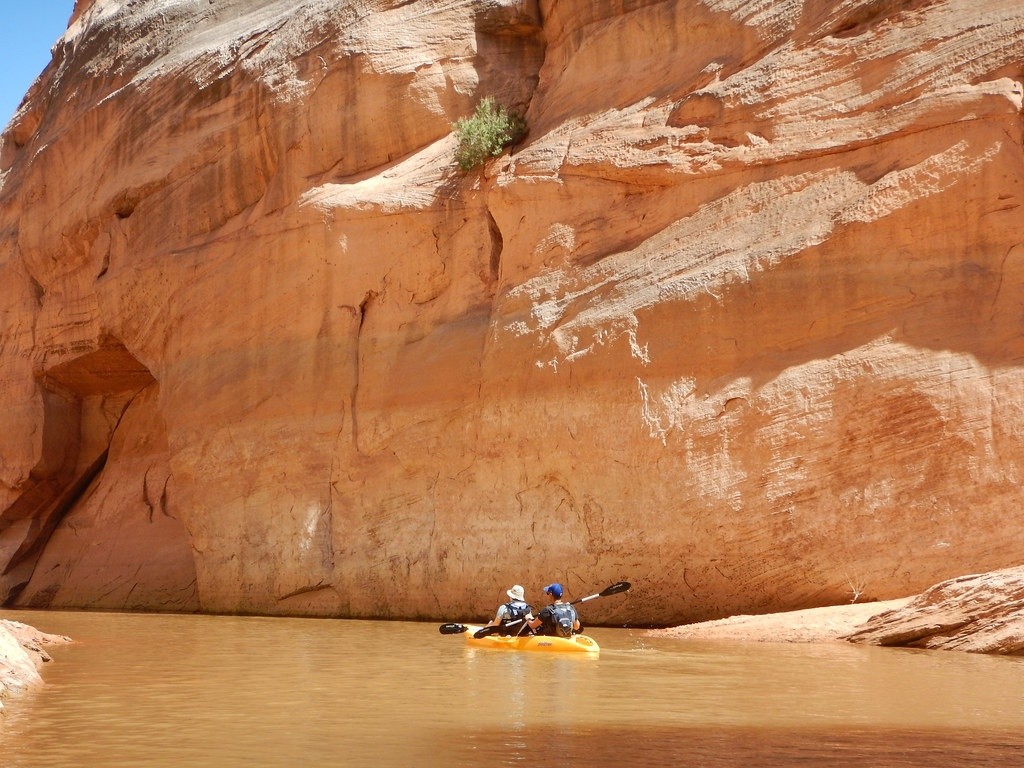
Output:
[507, 585, 524, 601]
[543, 583, 562, 597]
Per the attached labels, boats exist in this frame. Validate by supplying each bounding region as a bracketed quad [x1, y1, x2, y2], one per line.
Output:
[439, 623, 601, 652]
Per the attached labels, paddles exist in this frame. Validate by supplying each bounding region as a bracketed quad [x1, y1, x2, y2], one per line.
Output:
[439, 581, 631, 639]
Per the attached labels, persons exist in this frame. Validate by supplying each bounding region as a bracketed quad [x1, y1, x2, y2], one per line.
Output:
[526, 583, 580, 638]
[485, 584, 535, 636]
